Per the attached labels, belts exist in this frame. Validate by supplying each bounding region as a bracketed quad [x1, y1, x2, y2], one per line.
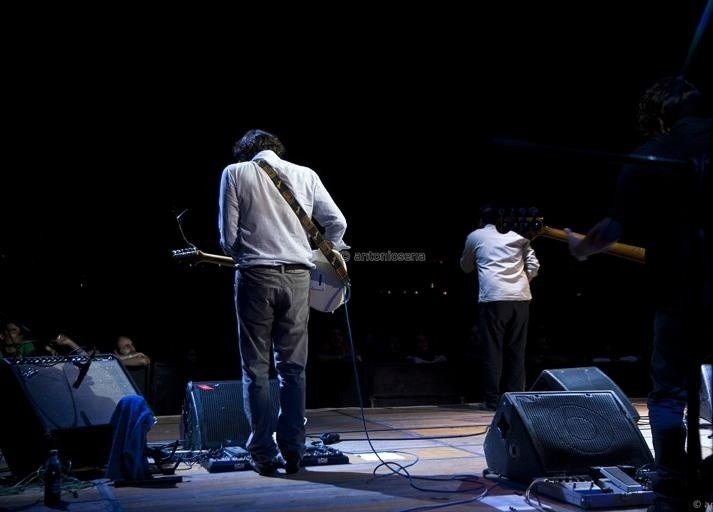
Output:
[245, 263, 311, 270]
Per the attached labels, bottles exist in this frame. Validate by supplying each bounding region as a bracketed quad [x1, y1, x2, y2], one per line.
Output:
[43, 449, 63, 509]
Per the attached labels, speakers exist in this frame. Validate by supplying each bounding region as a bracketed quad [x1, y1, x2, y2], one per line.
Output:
[698, 365, 713, 423]
[528, 367, 640, 422]
[483, 390, 655, 481]
[1, 354, 158, 481]
[179, 381, 251, 450]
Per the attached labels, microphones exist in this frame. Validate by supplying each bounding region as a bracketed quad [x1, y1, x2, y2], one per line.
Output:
[72, 346, 100, 389]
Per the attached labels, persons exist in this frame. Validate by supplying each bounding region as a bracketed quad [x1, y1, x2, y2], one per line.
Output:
[218, 128, 349, 476]
[0, 319, 34, 359]
[24, 333, 88, 356]
[460, 207, 543, 410]
[562, 73, 713, 495]
[103, 332, 153, 370]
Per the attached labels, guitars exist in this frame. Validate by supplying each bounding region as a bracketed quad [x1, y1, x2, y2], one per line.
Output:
[171, 246, 347, 313]
[477, 206, 644, 264]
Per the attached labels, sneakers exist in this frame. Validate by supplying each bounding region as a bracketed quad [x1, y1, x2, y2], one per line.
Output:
[249, 456, 280, 477]
[479, 400, 497, 411]
[284, 458, 301, 476]
